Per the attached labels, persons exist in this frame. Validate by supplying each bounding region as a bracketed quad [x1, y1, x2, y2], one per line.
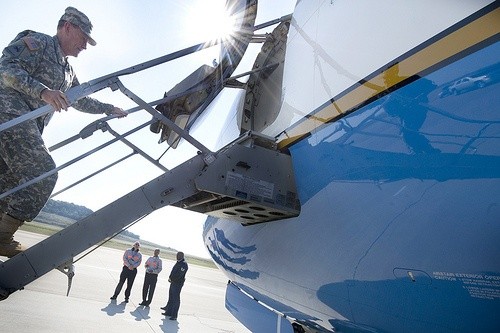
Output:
[110, 241, 142, 303]
[0, 7, 129, 258]
[138, 248, 163, 307]
[161, 252, 188, 320]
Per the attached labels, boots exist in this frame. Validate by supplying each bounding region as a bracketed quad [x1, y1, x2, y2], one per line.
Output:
[0, 214, 28, 257]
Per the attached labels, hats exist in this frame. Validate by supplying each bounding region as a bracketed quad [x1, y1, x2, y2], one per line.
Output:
[60, 6, 97, 47]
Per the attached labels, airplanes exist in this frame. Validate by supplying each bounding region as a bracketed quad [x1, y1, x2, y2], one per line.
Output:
[0, 1, 500, 333]
